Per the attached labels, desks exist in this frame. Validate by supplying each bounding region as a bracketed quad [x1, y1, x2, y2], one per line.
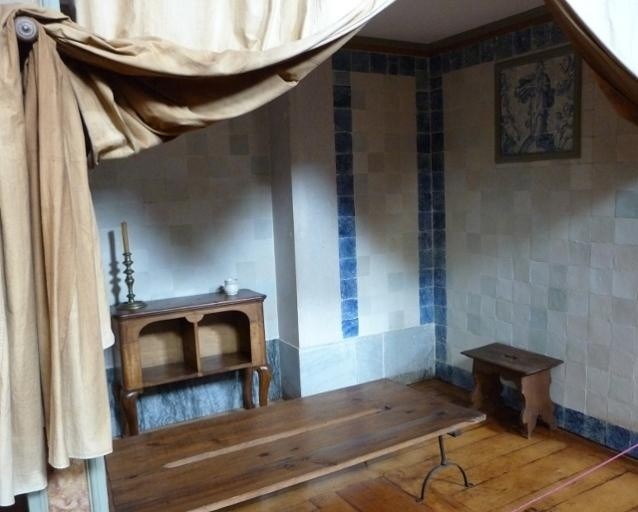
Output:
[104, 378, 487, 512]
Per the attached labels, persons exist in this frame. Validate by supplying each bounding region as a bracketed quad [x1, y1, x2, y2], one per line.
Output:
[501, 54, 573, 152]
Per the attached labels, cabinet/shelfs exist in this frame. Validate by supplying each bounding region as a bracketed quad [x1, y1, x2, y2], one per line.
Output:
[106, 288, 273, 438]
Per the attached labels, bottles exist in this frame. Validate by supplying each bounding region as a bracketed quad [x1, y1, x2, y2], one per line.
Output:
[223, 277, 239, 296]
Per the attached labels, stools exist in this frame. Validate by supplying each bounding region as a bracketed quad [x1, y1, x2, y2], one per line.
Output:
[460, 342, 564, 439]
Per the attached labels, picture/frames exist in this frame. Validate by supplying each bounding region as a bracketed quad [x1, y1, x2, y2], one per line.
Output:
[493, 43, 582, 164]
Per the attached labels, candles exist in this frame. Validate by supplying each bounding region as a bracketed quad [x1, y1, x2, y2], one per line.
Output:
[120, 218, 130, 253]
